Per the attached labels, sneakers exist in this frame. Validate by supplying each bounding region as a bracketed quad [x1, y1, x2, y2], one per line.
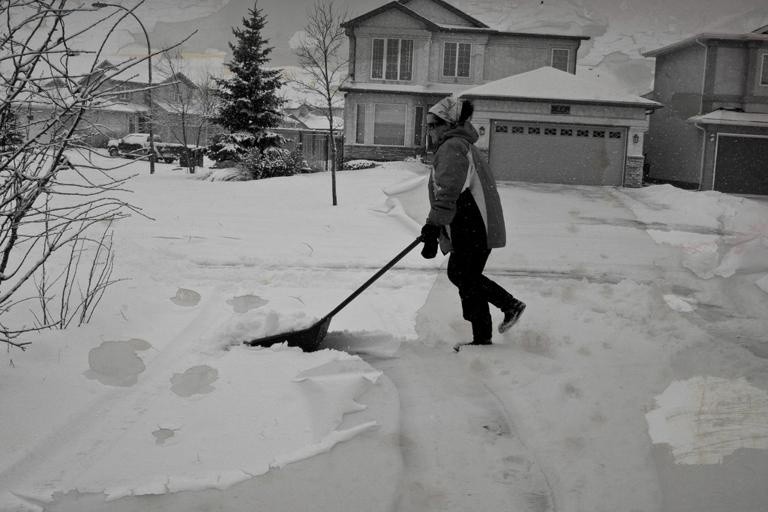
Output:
[452, 341, 492, 352]
[499, 299, 525, 330]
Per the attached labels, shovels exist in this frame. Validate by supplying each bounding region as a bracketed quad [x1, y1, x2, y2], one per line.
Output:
[251, 238, 422, 352]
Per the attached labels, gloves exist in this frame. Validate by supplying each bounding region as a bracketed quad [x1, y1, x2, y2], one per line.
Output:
[421, 223, 440, 242]
[420, 240, 438, 259]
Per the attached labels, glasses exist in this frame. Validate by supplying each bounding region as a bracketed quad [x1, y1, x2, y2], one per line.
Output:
[427, 121, 442, 131]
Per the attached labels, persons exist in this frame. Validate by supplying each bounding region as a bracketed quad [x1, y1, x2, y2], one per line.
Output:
[421, 96, 527, 353]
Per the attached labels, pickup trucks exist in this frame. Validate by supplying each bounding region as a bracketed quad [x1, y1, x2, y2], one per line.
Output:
[107, 133, 207, 167]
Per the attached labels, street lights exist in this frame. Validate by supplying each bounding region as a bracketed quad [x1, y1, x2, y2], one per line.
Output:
[92, 3, 154, 175]
